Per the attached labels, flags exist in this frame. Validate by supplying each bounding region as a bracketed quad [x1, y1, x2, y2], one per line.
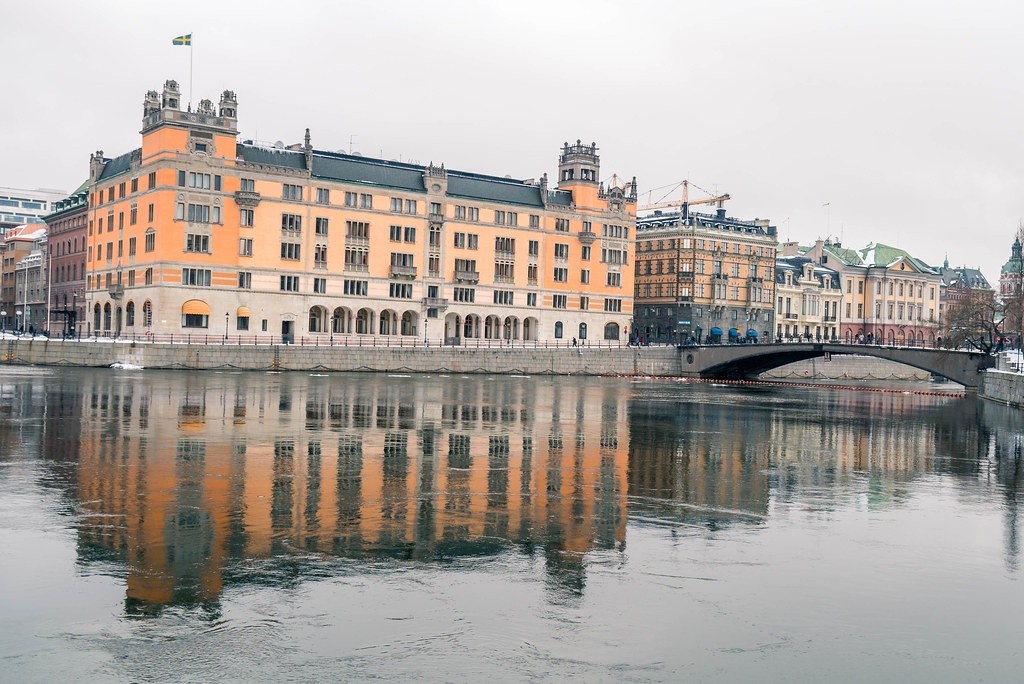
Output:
[173, 34, 191, 45]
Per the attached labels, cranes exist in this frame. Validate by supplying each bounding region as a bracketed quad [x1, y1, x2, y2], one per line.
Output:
[600, 173, 626, 187]
[637, 180, 731, 212]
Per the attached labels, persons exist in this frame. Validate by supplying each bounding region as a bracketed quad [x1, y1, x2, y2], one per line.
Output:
[807, 333, 813, 341]
[799, 335, 802, 343]
[705, 335, 713, 345]
[779, 335, 782, 342]
[688, 334, 701, 346]
[29, 324, 33, 334]
[789, 336, 793, 342]
[572, 337, 577, 347]
[859, 331, 872, 345]
[636, 337, 644, 347]
[19, 324, 23, 334]
[736, 336, 758, 344]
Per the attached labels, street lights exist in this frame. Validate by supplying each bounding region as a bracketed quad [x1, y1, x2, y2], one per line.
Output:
[16, 261, 28, 332]
[330, 316, 334, 345]
[1, 309, 7, 329]
[225, 312, 229, 339]
[41, 248, 51, 336]
[16, 311, 22, 330]
[581, 322, 586, 345]
[425, 318, 428, 347]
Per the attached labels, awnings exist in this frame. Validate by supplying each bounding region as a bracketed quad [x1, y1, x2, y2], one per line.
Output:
[747, 330, 757, 336]
[711, 327, 723, 335]
[729, 329, 740, 336]
